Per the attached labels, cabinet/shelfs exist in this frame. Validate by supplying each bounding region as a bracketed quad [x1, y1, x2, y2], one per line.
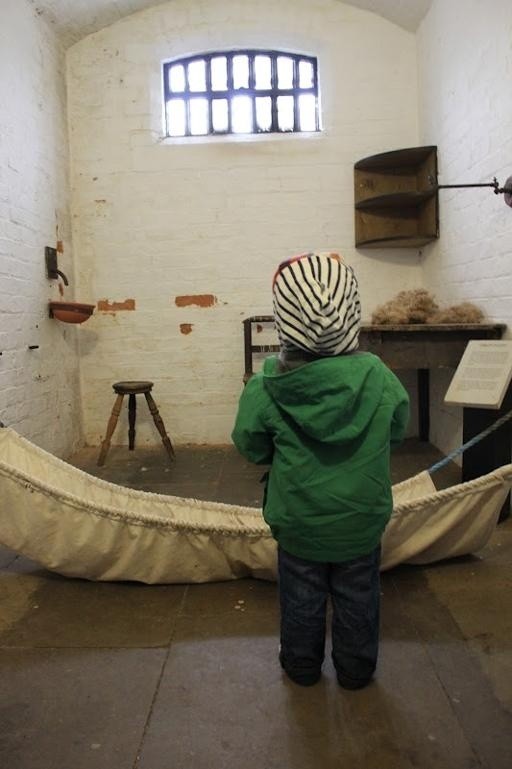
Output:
[353, 145, 441, 246]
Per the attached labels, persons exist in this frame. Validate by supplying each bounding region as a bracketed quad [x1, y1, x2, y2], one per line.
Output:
[230, 251, 413, 689]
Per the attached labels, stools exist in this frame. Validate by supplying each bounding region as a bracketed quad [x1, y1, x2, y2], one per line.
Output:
[93, 377, 182, 471]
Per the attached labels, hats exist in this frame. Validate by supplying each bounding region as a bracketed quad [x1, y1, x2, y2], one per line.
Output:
[272, 255, 362, 358]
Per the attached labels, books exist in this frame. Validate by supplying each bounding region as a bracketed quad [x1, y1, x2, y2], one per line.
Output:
[442, 338, 512, 410]
